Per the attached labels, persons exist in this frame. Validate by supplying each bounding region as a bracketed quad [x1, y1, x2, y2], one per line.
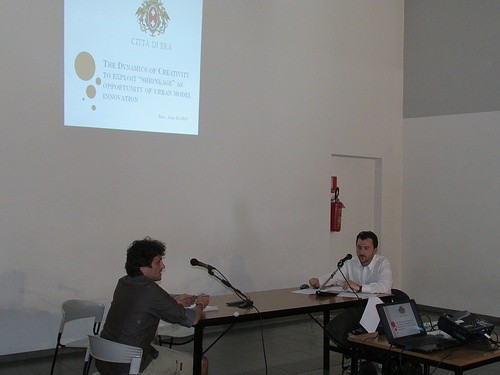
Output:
[95, 236, 209, 375]
[309, 231, 392, 373]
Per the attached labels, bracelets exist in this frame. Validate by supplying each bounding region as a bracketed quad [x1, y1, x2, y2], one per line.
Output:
[356, 285, 362, 293]
[197, 301, 204, 309]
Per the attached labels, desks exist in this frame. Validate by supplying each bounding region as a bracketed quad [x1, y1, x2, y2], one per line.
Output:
[345, 332, 500, 375]
[192, 285, 393, 375]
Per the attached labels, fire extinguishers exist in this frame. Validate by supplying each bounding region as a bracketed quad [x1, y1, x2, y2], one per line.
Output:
[330, 187, 345, 231]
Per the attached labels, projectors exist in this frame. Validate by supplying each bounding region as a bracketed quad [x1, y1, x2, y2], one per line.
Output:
[437, 310, 495, 343]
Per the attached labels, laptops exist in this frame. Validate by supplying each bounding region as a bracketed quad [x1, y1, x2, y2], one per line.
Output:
[375, 299, 458, 354]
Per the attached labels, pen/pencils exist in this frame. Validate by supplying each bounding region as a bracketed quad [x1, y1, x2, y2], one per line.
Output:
[192, 295, 209, 298]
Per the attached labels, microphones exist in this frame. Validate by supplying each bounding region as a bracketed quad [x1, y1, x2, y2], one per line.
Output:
[338, 254, 352, 267]
[190, 258, 215, 269]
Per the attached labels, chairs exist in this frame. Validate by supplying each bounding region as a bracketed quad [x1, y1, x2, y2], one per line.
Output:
[331, 288, 410, 375]
[86, 335, 143, 375]
[49, 300, 105, 375]
[155, 293, 195, 349]
